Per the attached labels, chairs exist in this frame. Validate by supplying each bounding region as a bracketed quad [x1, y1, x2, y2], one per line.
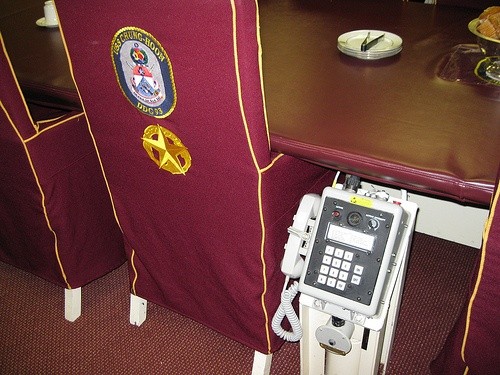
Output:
[52, 1, 343, 375]
[429, 166, 500, 374]
[0, 31, 127, 323]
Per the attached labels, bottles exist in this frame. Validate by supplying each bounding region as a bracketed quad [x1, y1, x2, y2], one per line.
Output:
[44, 0, 57, 22]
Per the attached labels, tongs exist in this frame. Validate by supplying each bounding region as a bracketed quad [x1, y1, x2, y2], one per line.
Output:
[360, 32, 385, 52]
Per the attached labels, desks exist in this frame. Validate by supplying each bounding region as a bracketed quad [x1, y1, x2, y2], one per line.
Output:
[0, 0, 500, 210]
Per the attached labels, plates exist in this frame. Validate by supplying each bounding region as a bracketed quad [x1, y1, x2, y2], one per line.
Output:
[36, 17, 59, 28]
[337, 30, 403, 60]
